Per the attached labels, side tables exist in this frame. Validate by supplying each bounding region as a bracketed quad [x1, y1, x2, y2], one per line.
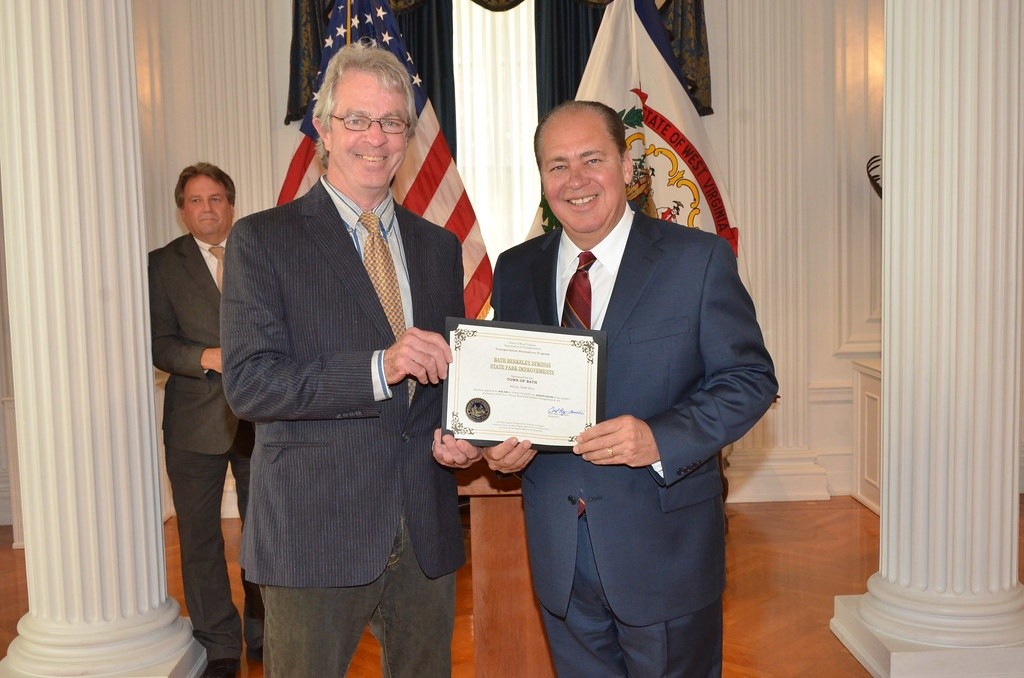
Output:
[1, 398, 25, 549]
[155, 372, 174, 523]
[851, 357, 882, 516]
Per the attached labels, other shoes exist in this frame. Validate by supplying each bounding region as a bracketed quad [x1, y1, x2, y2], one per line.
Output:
[201, 659, 241, 678]
[247, 646, 263, 660]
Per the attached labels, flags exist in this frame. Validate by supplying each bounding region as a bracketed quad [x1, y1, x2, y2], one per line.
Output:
[276, 0, 493, 321]
[526, 0, 749, 298]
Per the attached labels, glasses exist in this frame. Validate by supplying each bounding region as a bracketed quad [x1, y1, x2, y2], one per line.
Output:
[328, 114, 411, 134]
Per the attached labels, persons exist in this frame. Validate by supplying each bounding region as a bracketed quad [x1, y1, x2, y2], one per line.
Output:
[222, 44, 481, 677]
[149, 164, 265, 678]
[482, 101, 778, 677]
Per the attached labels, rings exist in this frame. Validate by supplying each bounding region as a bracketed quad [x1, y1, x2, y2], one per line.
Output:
[608, 446, 615, 458]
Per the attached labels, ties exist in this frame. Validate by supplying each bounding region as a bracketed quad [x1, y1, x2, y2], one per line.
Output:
[561, 252, 597, 516]
[209, 247, 225, 289]
[358, 212, 417, 404]
